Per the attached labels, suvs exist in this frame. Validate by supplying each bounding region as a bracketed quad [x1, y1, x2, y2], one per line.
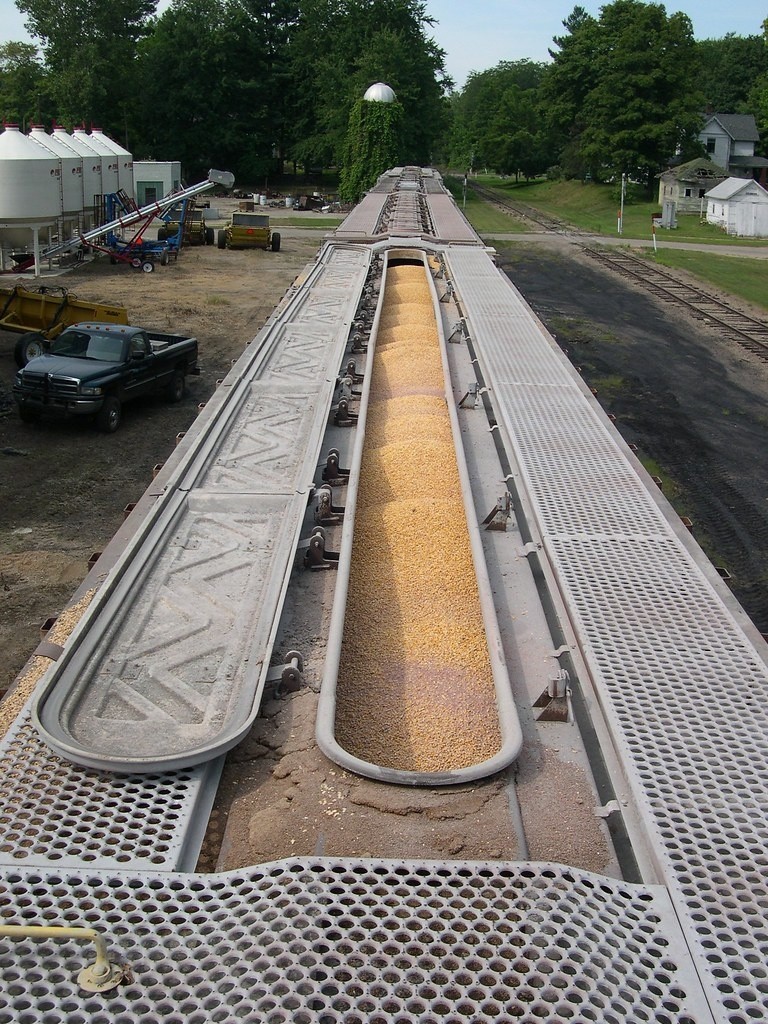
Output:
[217, 212, 280, 253]
[158, 208, 214, 246]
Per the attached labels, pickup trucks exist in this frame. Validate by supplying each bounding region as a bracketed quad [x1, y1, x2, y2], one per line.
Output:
[12, 321, 200, 435]
